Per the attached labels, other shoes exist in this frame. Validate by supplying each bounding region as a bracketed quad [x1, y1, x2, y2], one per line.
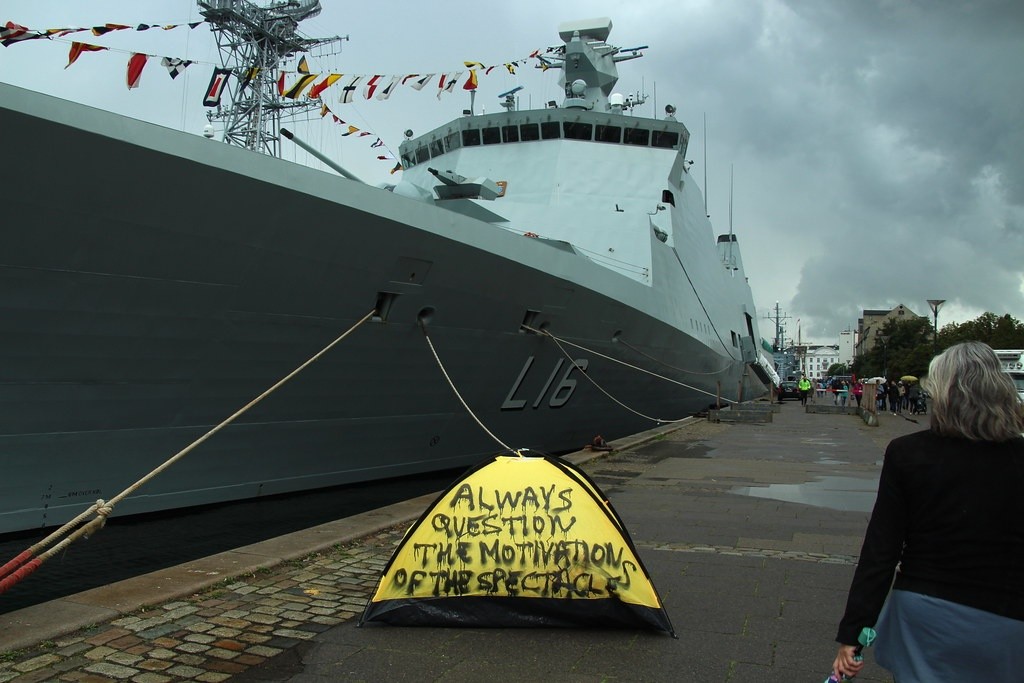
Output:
[878, 413, 880, 415]
[894, 413, 896, 416]
[910, 413, 911, 415]
[890, 412, 892, 415]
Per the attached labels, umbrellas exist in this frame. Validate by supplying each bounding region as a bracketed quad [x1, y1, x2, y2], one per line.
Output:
[900, 376, 918, 382]
[868, 377, 886, 384]
[824, 628, 877, 683]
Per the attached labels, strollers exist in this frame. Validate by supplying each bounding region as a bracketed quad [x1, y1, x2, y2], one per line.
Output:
[915, 395, 927, 415]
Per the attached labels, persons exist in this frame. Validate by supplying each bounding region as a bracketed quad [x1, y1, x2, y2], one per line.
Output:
[799, 374, 811, 406]
[834, 342, 1024, 683]
[810, 376, 864, 409]
[875, 380, 922, 416]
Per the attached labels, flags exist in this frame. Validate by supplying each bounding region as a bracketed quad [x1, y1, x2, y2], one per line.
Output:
[203, 66, 232, 107]
[321, 104, 403, 175]
[340, 77, 363, 103]
[126, 52, 150, 91]
[278, 72, 284, 95]
[284, 74, 320, 99]
[65, 41, 108, 70]
[0, 21, 202, 48]
[161, 57, 192, 79]
[363, 74, 461, 100]
[464, 49, 547, 90]
[239, 68, 261, 91]
[312, 74, 343, 97]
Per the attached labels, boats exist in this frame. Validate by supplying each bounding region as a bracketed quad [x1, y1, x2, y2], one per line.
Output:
[0, 1, 800, 541]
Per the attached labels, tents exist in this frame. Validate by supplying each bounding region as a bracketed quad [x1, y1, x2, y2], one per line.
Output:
[356, 448, 680, 640]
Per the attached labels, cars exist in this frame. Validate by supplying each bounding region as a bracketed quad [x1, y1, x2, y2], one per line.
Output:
[778, 381, 800, 400]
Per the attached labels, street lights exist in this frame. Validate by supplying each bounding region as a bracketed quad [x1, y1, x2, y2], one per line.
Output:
[927, 299, 946, 353]
